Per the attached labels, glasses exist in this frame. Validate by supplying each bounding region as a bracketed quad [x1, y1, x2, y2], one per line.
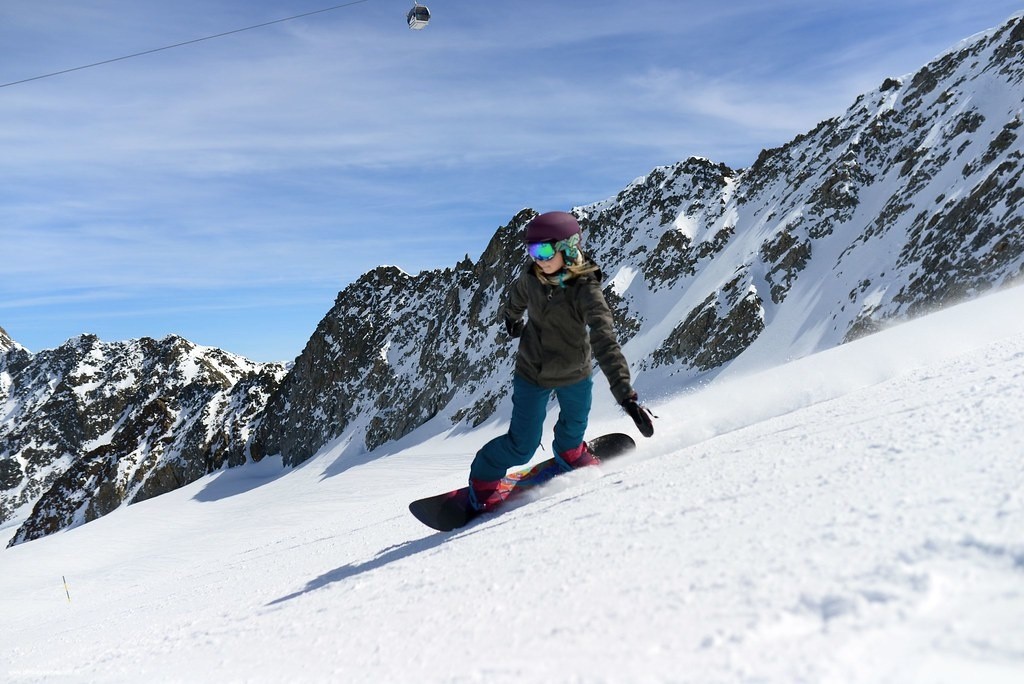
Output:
[526, 241, 556, 261]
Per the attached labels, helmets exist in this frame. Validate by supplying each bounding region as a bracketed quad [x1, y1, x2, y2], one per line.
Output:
[519, 211, 582, 244]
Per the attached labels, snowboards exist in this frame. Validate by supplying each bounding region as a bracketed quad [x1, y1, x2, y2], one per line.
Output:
[409, 431, 635, 532]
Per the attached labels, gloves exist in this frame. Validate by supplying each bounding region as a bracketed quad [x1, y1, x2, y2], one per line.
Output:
[505, 316, 527, 337]
[621, 396, 659, 438]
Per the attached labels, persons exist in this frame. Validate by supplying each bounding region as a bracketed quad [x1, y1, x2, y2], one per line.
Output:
[468, 212, 654, 514]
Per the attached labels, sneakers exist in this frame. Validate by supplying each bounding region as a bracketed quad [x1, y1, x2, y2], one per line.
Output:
[552, 440, 601, 472]
[467, 476, 506, 514]
